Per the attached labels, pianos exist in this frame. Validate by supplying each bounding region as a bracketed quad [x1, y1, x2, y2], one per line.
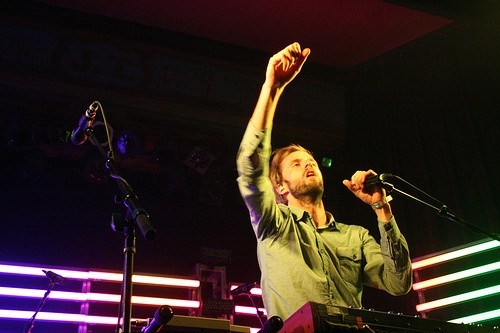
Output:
[279, 301, 500, 333]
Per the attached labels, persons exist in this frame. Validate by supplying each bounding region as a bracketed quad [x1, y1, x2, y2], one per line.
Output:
[236, 41, 413, 324]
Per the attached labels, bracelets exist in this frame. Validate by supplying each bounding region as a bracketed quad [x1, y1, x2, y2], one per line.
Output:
[371, 195, 393, 211]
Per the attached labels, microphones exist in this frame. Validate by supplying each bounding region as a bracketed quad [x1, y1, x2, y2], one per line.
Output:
[231, 278, 260, 295]
[364, 174, 392, 187]
[71, 101, 98, 146]
